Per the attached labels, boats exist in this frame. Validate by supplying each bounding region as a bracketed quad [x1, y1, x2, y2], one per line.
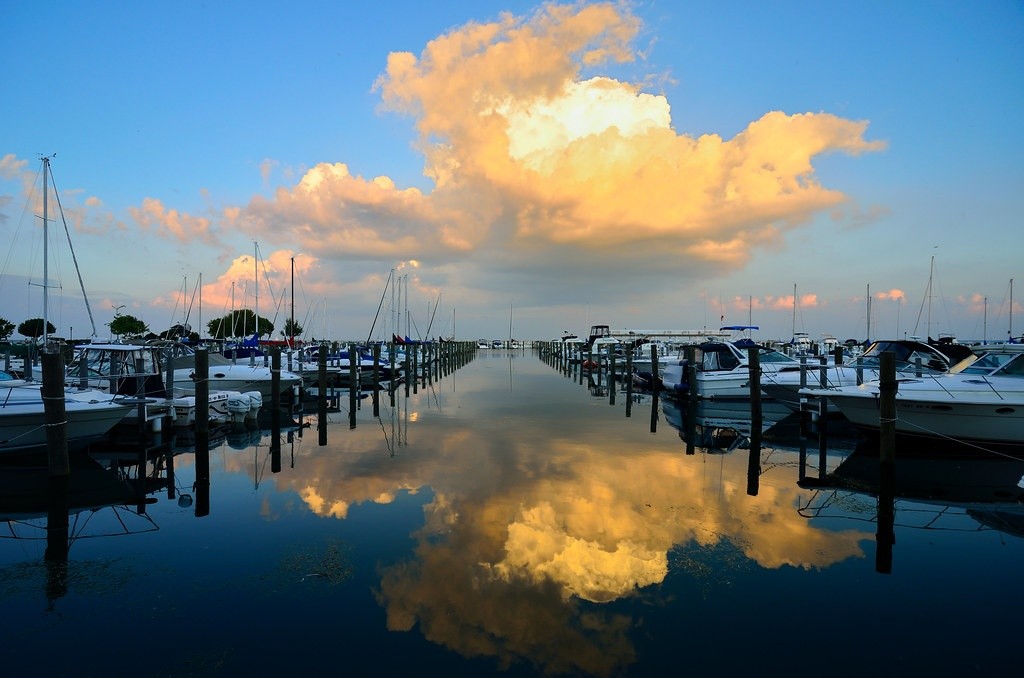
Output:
[1, 148, 1024, 507]
